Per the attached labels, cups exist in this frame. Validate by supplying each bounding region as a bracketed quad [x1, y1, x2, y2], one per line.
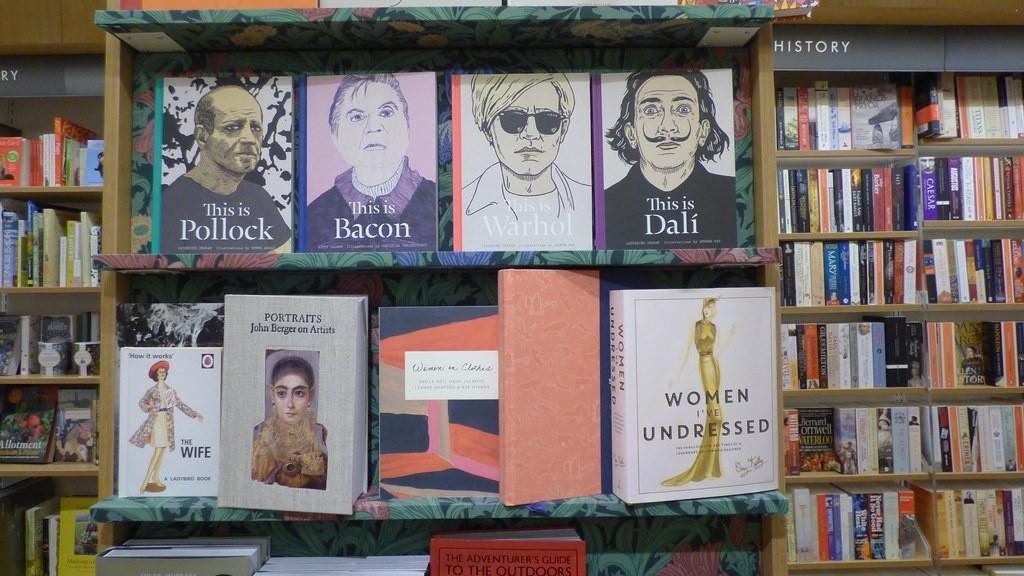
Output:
[74, 341, 100, 377]
[38, 341, 71, 378]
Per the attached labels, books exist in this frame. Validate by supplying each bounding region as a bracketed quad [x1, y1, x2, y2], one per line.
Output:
[609, 286, 780, 506]
[215, 295, 370, 516]
[780, 239, 1023, 307]
[0, 118, 104, 186]
[0, 200, 101, 288]
[151, 75, 296, 254]
[451, 73, 593, 253]
[0, 477, 98, 576]
[113, 302, 226, 500]
[783, 398, 1024, 476]
[591, 68, 738, 251]
[298, 71, 439, 254]
[95, 525, 586, 576]
[497, 268, 644, 507]
[378, 305, 499, 502]
[777, 155, 1024, 234]
[786, 480, 1024, 563]
[776, 70, 1024, 150]
[0, 384, 99, 467]
[780, 315, 1024, 391]
[0, 312, 100, 377]
